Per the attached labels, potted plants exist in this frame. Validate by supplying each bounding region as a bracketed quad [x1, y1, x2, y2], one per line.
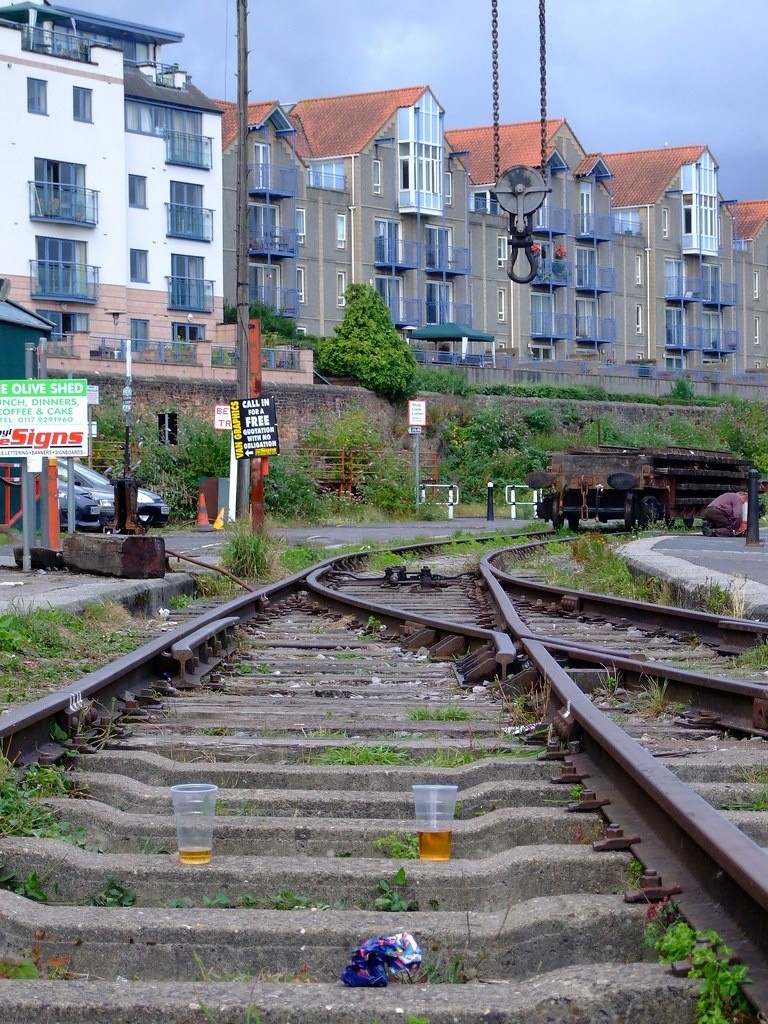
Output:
[76, 203, 85, 221]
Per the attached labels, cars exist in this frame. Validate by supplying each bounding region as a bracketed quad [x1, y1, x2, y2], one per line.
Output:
[57, 458, 171, 528]
[57, 479, 100, 530]
[81, 486, 115, 521]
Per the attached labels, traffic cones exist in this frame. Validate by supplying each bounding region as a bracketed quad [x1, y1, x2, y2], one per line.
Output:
[191, 492, 215, 532]
[212, 508, 226, 530]
[738, 497, 748, 534]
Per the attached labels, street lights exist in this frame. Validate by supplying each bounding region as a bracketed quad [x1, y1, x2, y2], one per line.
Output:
[105, 311, 127, 359]
[400, 326, 417, 349]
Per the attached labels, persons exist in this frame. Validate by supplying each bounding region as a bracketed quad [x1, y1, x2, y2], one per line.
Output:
[701, 491, 748, 537]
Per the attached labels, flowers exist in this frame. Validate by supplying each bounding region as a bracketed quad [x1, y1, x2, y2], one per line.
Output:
[532, 244, 541, 256]
[555, 246, 566, 258]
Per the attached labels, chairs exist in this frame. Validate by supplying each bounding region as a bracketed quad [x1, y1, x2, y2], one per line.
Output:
[265, 231, 288, 249]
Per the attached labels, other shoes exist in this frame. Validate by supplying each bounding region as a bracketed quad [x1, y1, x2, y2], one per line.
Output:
[702, 523, 716, 537]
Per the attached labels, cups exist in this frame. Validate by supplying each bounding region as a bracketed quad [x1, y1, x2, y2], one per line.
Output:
[170, 784, 219, 864]
[412, 784, 459, 861]
